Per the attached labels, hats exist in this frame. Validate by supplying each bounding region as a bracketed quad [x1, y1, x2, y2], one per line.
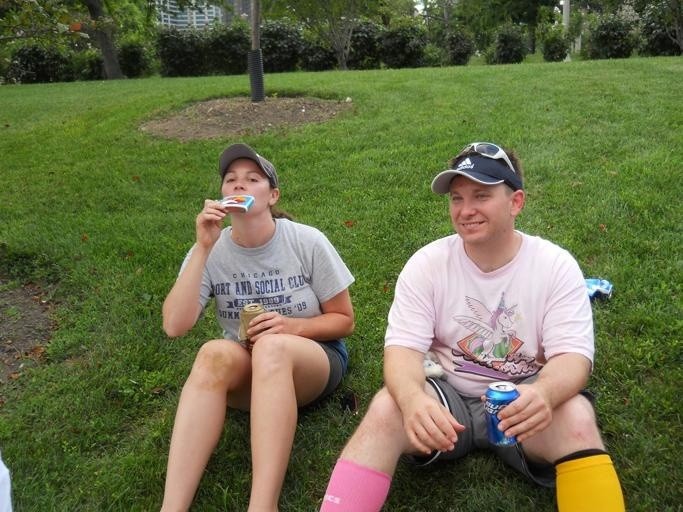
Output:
[430, 156, 522, 194]
[219, 143, 278, 187]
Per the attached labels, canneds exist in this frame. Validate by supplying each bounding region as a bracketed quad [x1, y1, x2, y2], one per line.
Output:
[600, 284, 613, 298]
[237, 303, 266, 352]
[484, 381, 520, 448]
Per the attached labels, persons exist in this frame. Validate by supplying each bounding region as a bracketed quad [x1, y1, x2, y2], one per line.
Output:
[319, 143, 625, 512]
[160, 142, 355, 512]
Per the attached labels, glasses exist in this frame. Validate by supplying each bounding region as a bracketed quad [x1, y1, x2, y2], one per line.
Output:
[454, 142, 516, 174]
